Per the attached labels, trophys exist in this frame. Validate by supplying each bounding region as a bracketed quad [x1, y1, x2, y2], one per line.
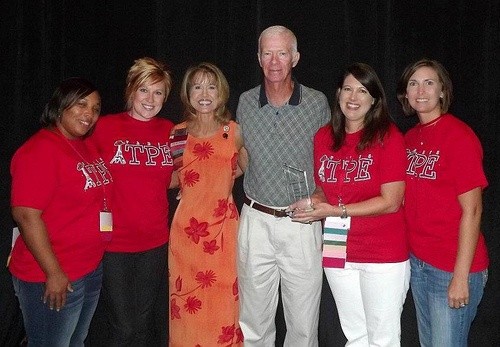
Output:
[283, 163, 314, 218]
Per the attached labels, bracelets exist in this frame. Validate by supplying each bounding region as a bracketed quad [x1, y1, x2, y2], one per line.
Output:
[340, 205, 347, 218]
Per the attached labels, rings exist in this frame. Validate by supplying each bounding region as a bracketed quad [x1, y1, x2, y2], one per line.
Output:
[460, 303, 465, 307]
[308, 219, 312, 225]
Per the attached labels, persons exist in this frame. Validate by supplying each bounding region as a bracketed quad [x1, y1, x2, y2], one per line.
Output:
[168, 63, 249, 347]
[84, 58, 179, 347]
[283, 62, 412, 347]
[396, 59, 489, 347]
[236, 26, 332, 347]
[7, 77, 116, 347]
